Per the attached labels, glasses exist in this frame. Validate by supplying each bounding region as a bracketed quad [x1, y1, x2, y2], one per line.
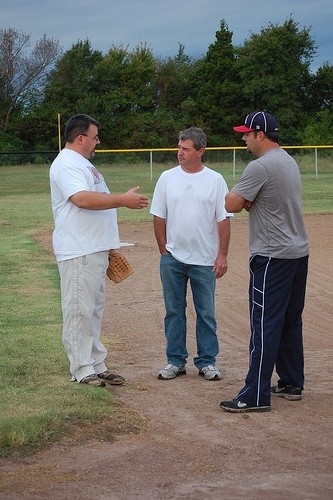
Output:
[78, 134, 99, 142]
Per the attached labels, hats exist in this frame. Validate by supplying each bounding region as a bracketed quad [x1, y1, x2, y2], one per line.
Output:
[232, 110, 280, 133]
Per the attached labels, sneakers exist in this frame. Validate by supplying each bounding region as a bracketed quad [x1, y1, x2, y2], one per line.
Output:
[220, 399, 272, 413]
[270, 384, 303, 401]
[157, 364, 187, 380]
[97, 370, 126, 385]
[198, 365, 223, 380]
[80, 374, 106, 387]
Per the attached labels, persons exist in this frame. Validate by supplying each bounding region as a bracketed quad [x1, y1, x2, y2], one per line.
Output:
[220, 112, 308, 412]
[149, 126, 233, 380]
[50, 114, 149, 390]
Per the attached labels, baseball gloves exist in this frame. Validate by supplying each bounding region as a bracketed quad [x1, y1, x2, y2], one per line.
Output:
[107, 250, 135, 283]
